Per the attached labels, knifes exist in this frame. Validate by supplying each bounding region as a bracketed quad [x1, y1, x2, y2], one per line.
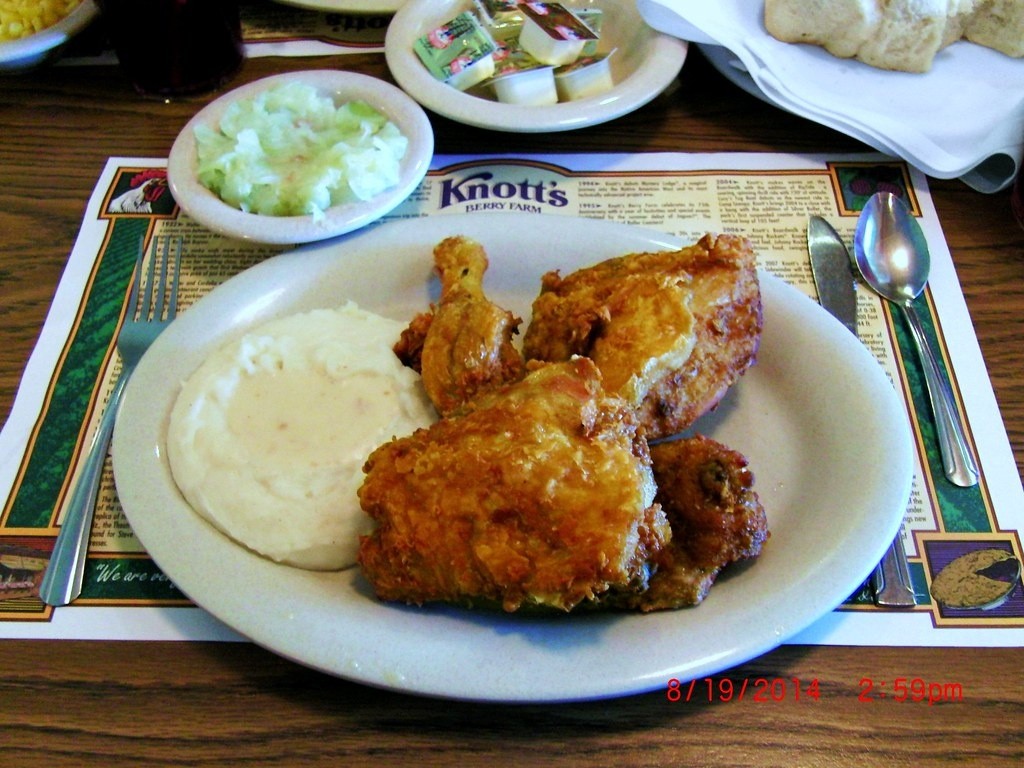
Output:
[806, 213, 918, 607]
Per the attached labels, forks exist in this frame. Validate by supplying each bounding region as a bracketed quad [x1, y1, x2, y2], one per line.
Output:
[36, 231, 188, 608]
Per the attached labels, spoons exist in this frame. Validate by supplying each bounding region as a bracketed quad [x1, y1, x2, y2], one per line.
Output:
[855, 189, 980, 493]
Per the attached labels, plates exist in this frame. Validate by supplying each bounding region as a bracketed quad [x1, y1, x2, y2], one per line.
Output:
[168, 67, 435, 247]
[384, 1, 691, 131]
[1, 1, 105, 65]
[110, 208, 912, 701]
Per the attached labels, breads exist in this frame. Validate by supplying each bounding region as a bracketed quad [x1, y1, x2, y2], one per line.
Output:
[763, 0, 1024, 75]
